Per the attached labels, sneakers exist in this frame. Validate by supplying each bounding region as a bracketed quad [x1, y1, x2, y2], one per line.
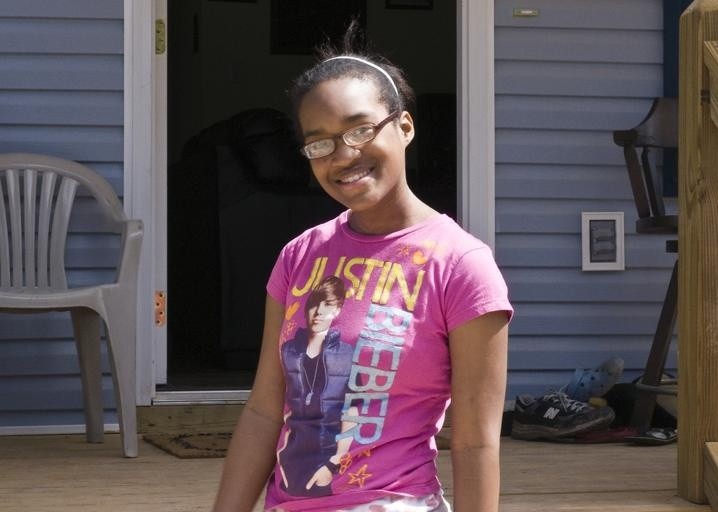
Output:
[562, 355, 626, 404]
[510, 391, 617, 444]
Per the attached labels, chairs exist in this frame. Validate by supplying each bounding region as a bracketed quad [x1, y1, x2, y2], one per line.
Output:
[613, 93, 680, 429]
[0, 150, 148, 458]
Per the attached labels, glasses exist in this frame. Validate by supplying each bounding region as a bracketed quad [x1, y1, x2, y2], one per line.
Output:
[297, 109, 403, 161]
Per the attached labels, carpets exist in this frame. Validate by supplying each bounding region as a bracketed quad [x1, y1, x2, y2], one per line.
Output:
[140, 427, 453, 459]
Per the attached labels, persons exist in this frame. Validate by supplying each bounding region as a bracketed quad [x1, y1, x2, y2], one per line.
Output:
[213, 38, 515, 512]
[277, 275, 367, 497]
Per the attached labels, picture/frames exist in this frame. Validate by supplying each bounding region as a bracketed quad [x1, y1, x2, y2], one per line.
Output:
[579, 209, 626, 273]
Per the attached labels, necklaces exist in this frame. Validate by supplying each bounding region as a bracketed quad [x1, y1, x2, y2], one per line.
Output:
[304, 355, 320, 405]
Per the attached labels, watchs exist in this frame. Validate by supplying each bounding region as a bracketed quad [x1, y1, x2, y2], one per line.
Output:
[325, 460, 341, 477]
